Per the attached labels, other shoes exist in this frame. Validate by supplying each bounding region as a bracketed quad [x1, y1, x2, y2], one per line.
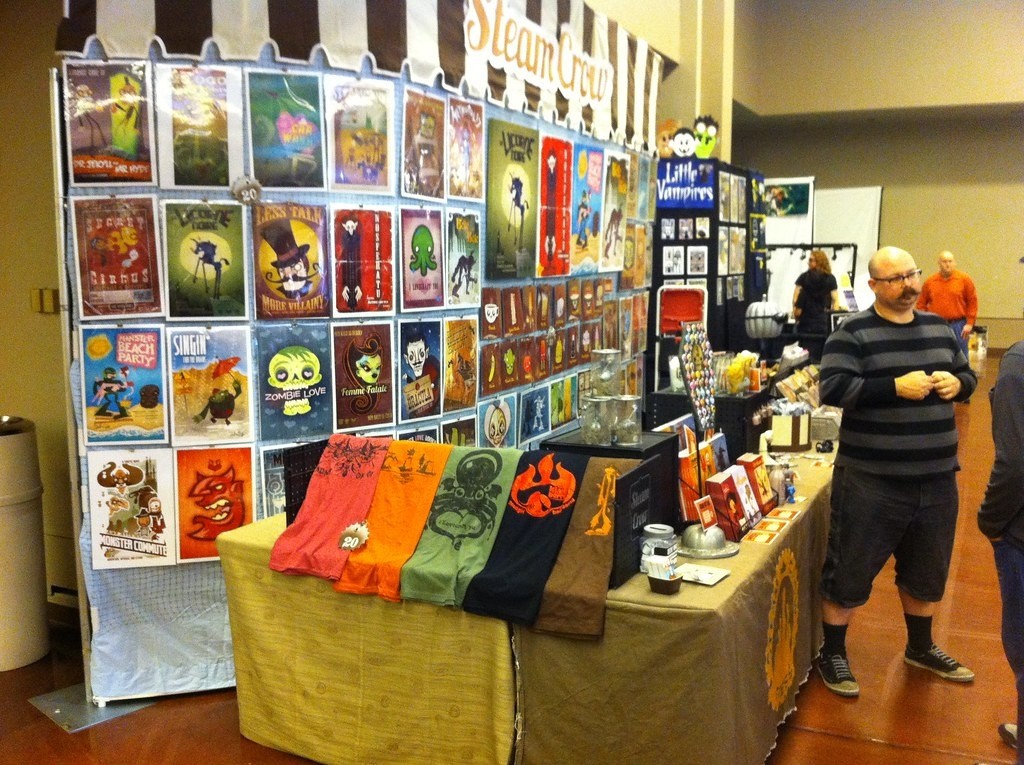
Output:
[997, 722, 1017, 749]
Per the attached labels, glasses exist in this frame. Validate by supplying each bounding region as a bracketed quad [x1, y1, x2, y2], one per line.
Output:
[868, 268, 924, 288]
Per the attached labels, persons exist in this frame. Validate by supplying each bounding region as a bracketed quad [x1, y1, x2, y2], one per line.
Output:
[976, 341, 1023, 765]
[915, 251, 978, 403]
[819, 247, 978, 696]
[794, 249, 839, 335]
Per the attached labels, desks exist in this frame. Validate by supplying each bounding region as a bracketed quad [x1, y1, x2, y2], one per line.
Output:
[216, 406, 841, 765]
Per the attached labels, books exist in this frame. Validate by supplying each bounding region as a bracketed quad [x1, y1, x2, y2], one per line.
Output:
[652, 411, 773, 542]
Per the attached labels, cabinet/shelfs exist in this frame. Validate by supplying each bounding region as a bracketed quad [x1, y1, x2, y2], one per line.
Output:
[646, 157, 750, 432]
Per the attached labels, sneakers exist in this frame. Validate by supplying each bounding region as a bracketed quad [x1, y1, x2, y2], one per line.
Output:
[903, 643, 975, 683]
[814, 649, 860, 696]
